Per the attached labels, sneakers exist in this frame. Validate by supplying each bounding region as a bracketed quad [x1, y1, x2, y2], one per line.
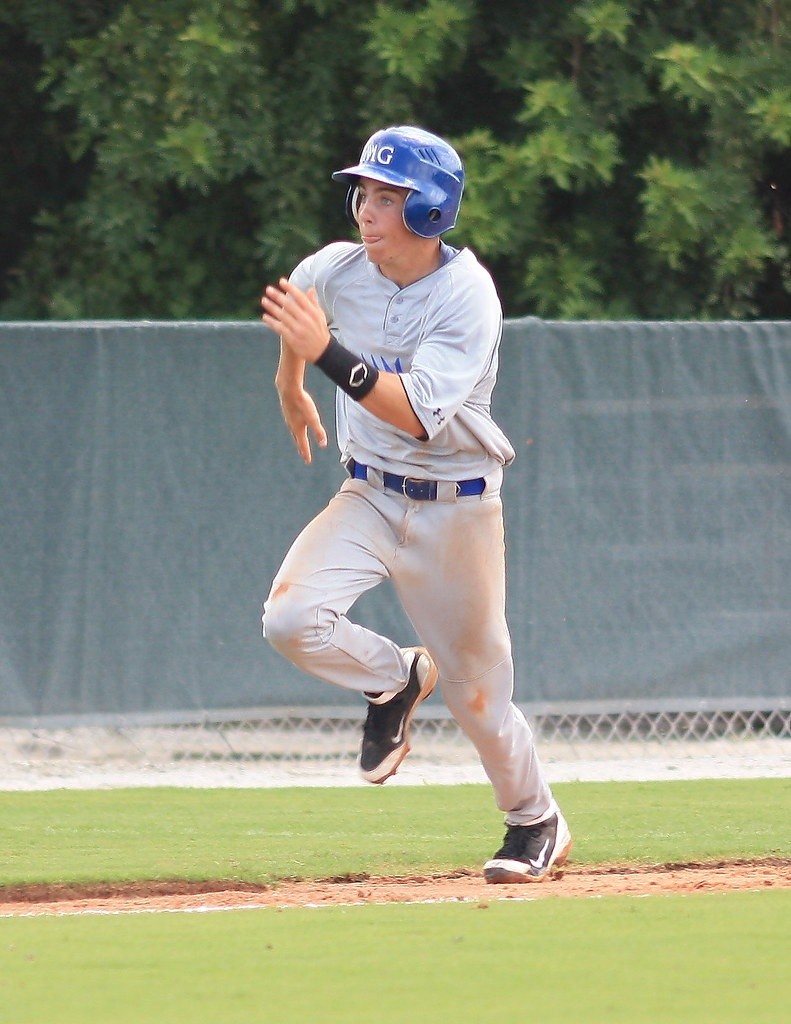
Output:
[355, 647, 439, 784]
[484, 798, 573, 883]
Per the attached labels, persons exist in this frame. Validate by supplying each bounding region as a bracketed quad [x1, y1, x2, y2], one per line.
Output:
[262, 127, 570, 883]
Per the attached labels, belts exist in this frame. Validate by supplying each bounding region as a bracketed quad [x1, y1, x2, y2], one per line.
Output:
[351, 459, 485, 502]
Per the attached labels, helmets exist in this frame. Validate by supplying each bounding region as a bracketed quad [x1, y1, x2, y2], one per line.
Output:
[331, 125, 465, 239]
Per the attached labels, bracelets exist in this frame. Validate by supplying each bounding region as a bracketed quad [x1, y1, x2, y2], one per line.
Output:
[313, 336, 379, 401]
